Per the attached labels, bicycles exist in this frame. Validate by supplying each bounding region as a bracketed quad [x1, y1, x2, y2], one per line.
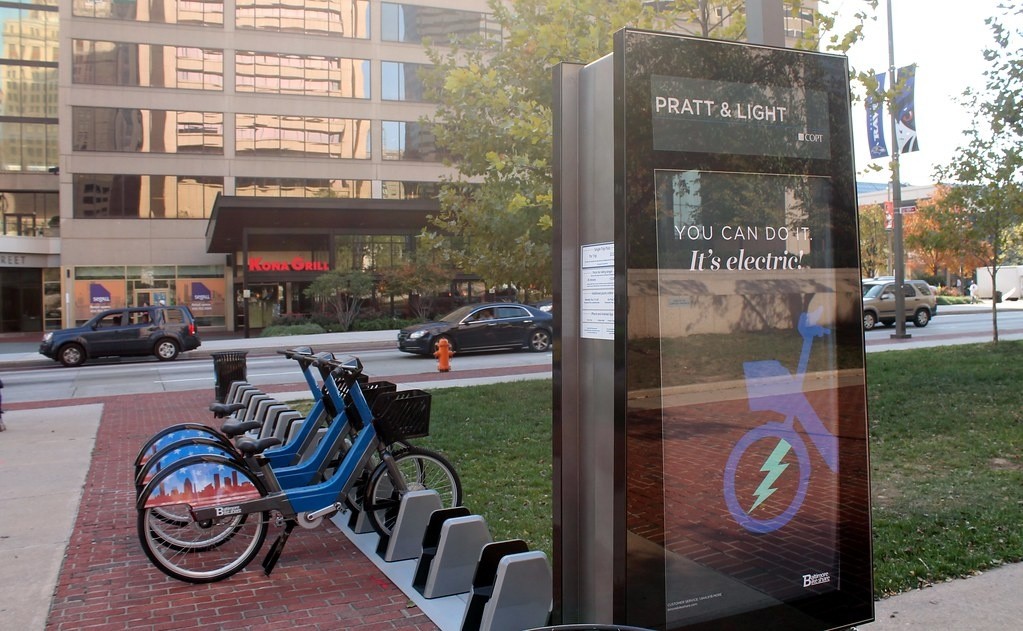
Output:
[130, 343, 463, 583]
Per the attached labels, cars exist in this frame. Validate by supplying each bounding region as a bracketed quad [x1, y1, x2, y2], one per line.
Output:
[529, 301, 554, 315]
[863, 276, 895, 294]
[398, 300, 554, 356]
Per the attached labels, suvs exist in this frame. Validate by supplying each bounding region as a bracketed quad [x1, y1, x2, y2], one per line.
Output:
[861, 280, 937, 331]
[38, 306, 202, 367]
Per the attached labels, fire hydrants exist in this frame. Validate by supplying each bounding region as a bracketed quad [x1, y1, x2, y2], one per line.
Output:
[432, 337, 456, 372]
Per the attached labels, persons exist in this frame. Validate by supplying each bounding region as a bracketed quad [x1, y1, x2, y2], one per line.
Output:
[969, 280, 978, 304]
[112, 317, 120, 325]
[478, 310, 493, 320]
[159, 300, 165, 306]
[142, 314, 148, 323]
[144, 302, 149, 314]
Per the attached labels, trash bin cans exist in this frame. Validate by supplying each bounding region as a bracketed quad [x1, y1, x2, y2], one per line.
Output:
[209, 349, 248, 404]
